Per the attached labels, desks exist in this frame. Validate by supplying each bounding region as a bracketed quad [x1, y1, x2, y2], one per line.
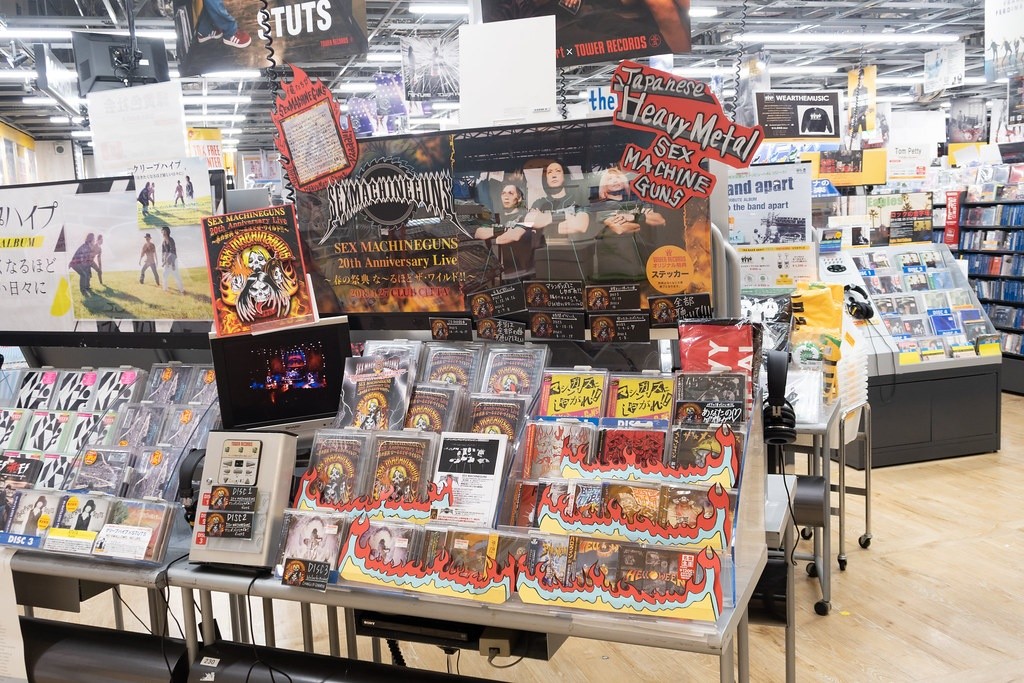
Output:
[10, 370, 872, 682]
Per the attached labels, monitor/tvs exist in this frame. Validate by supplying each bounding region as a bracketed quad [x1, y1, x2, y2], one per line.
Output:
[209, 315, 353, 429]
[70, 30, 170, 99]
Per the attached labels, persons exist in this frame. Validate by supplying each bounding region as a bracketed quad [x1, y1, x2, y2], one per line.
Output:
[525, 158, 593, 279]
[0, 483, 12, 522]
[892, 322, 903, 335]
[913, 324, 924, 335]
[595, 168, 667, 276]
[19, 496, 47, 536]
[70, 232, 104, 292]
[173, 180, 185, 207]
[70, 499, 96, 530]
[184, 175, 194, 199]
[138, 233, 160, 286]
[138, 181, 155, 216]
[161, 227, 185, 293]
[474, 183, 532, 272]
[197, 0, 251, 48]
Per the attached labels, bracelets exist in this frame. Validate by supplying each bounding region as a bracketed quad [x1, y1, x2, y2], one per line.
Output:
[551, 211, 565, 222]
[493, 227, 504, 238]
[632, 213, 646, 223]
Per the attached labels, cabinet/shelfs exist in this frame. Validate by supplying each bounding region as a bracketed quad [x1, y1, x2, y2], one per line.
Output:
[820, 201, 1024, 470]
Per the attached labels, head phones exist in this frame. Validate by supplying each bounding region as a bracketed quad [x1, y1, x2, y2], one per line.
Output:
[844, 285, 874, 319]
[178, 448, 207, 528]
[763, 351, 797, 445]
